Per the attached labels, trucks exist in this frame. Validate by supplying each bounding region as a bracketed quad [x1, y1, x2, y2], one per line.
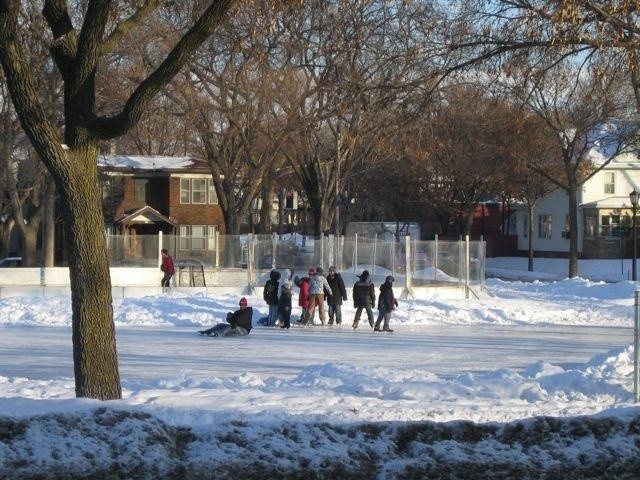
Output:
[344, 221, 427, 274]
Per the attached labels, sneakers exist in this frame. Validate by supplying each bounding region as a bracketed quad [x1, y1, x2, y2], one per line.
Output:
[208, 331, 218, 337]
[198, 331, 208, 336]
[352, 323, 357, 328]
[375, 326, 382, 332]
[370, 323, 375, 327]
[383, 329, 393, 333]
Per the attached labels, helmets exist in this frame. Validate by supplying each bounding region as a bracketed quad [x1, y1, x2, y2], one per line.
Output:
[386, 276, 394, 285]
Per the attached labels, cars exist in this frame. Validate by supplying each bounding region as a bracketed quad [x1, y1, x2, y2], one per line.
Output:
[240, 231, 315, 264]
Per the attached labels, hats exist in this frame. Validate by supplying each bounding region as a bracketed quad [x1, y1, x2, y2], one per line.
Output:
[240, 298, 247, 306]
[284, 279, 292, 288]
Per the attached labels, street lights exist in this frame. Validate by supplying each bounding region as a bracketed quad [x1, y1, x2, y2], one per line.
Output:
[628, 188, 640, 280]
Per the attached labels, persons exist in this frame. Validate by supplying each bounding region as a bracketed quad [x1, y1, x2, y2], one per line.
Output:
[305, 269, 334, 325]
[278, 282, 293, 329]
[350, 269, 376, 327]
[262, 270, 280, 325]
[159, 248, 175, 288]
[375, 276, 399, 332]
[293, 267, 317, 323]
[198, 298, 253, 338]
[324, 264, 347, 325]
